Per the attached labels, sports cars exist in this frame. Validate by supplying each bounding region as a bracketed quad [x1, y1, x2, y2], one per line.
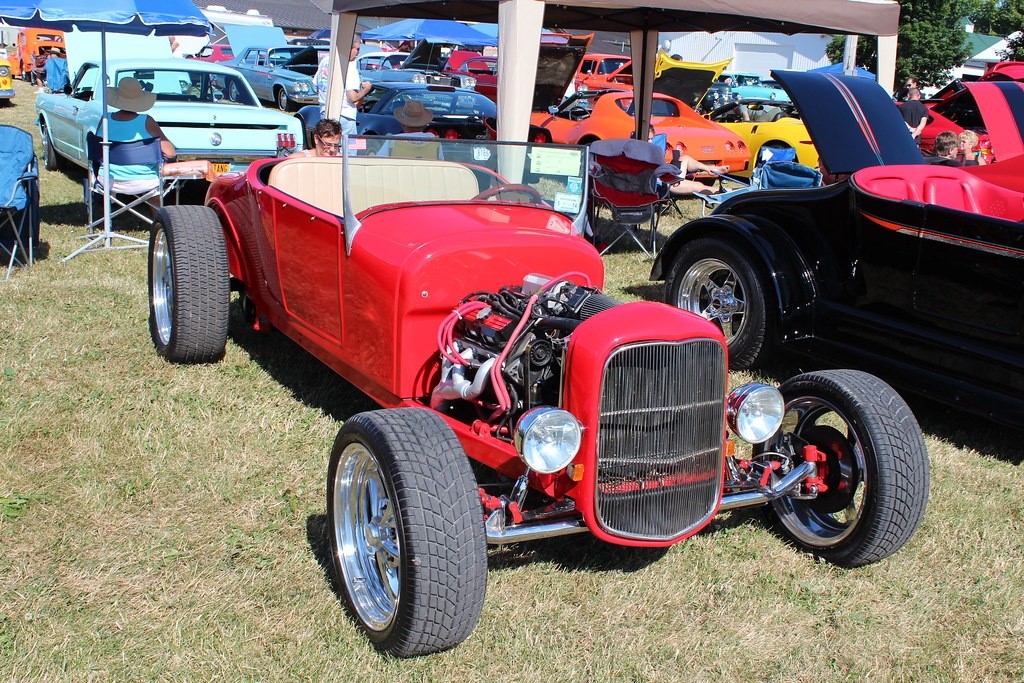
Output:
[655, 47, 820, 178]
[528, 31, 750, 186]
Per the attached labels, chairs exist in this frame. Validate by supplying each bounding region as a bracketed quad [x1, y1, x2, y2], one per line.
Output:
[586, 134, 823, 258]
[390, 135, 440, 160]
[0, 124, 40, 279]
[85, 131, 163, 237]
[37, 57, 66, 92]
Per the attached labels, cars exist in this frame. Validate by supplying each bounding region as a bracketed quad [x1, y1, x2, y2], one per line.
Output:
[571, 52, 798, 122]
[146, 133, 932, 658]
[649, 69, 1023, 447]
[1, 28, 67, 101]
[34, 57, 303, 210]
[182, 29, 498, 113]
[291, 81, 554, 191]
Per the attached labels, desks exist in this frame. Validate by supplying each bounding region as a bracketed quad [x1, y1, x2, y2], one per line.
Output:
[162, 160, 210, 205]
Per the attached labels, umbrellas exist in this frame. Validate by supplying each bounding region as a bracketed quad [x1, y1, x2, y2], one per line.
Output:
[0, 0, 214, 251]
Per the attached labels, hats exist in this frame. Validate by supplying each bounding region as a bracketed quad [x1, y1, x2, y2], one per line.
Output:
[394, 100, 433, 127]
[107, 77, 157, 112]
[46, 48, 62, 55]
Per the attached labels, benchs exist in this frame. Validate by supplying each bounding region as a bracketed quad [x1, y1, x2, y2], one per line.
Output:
[852, 165, 1024, 224]
[268, 156, 480, 218]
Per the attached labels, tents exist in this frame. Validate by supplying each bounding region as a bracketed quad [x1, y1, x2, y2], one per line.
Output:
[361, 18, 498, 46]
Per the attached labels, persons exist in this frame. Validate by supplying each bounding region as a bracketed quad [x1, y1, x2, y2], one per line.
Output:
[33, 47, 61, 87]
[287, 119, 342, 158]
[169, 36, 182, 58]
[898, 88, 929, 148]
[376, 100, 445, 161]
[959, 129, 986, 166]
[733, 104, 772, 121]
[906, 77, 925, 99]
[930, 131, 959, 160]
[312, 33, 372, 157]
[658, 155, 732, 197]
[96, 77, 176, 216]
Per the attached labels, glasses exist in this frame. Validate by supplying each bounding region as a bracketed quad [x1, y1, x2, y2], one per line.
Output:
[317, 135, 342, 149]
[352, 46, 360, 51]
[906, 84, 913, 88]
